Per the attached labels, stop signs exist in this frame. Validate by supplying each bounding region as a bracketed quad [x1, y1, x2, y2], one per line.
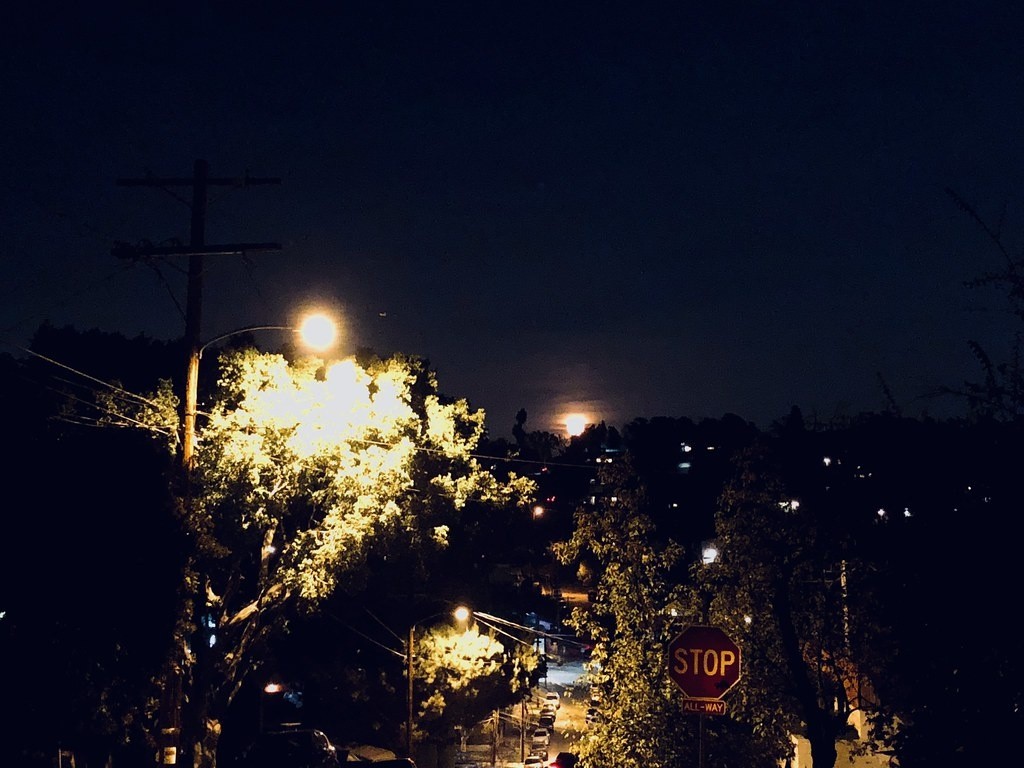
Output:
[667, 624, 741, 700]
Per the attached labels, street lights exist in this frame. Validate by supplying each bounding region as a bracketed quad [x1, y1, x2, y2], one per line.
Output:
[183, 310, 339, 765]
[406, 606, 469, 757]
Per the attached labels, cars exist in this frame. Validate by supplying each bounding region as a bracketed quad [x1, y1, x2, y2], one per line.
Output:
[531, 728, 550, 747]
[589, 683, 602, 706]
[528, 742, 548, 761]
[241, 728, 338, 768]
[524, 756, 544, 768]
[585, 709, 598, 725]
[536, 691, 560, 733]
